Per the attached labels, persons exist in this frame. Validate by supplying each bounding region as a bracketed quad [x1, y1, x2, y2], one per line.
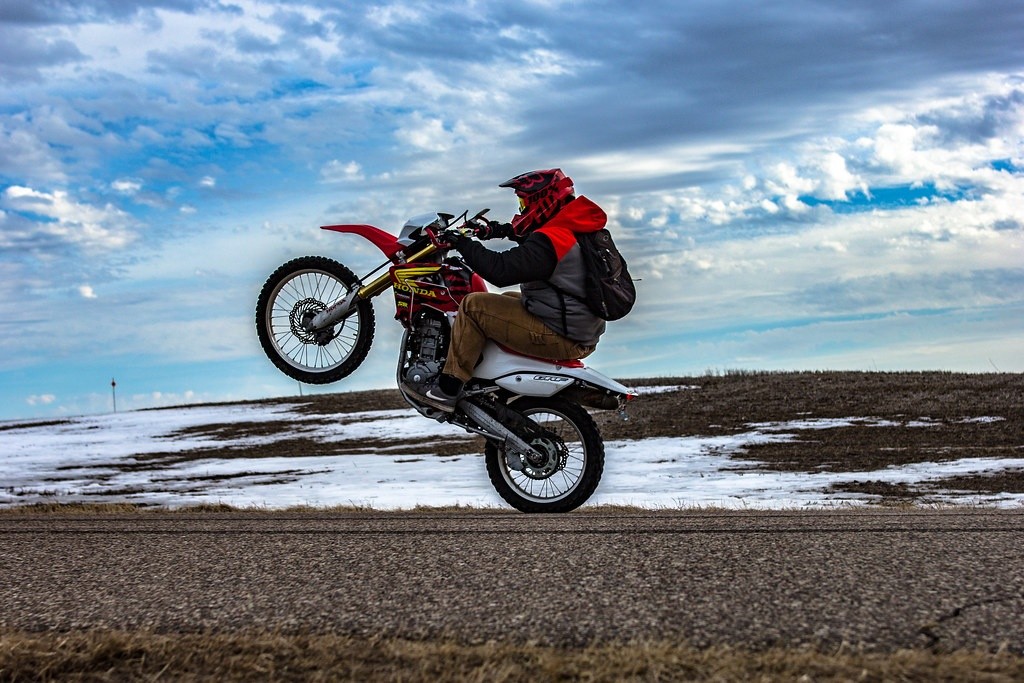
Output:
[397, 167, 609, 413]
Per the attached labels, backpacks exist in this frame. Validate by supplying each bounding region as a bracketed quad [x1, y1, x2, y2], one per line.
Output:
[543, 230, 636, 335]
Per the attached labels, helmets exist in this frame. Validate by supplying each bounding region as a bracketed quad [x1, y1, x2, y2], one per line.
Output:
[499, 169, 574, 237]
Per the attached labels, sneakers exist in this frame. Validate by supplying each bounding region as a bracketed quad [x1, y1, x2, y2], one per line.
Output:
[401, 381, 457, 412]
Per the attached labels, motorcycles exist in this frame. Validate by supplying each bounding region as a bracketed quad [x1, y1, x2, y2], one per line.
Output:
[256, 208, 641, 514]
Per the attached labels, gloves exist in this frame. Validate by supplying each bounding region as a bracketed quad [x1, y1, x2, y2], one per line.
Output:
[440, 230, 461, 243]
[488, 221, 503, 240]
